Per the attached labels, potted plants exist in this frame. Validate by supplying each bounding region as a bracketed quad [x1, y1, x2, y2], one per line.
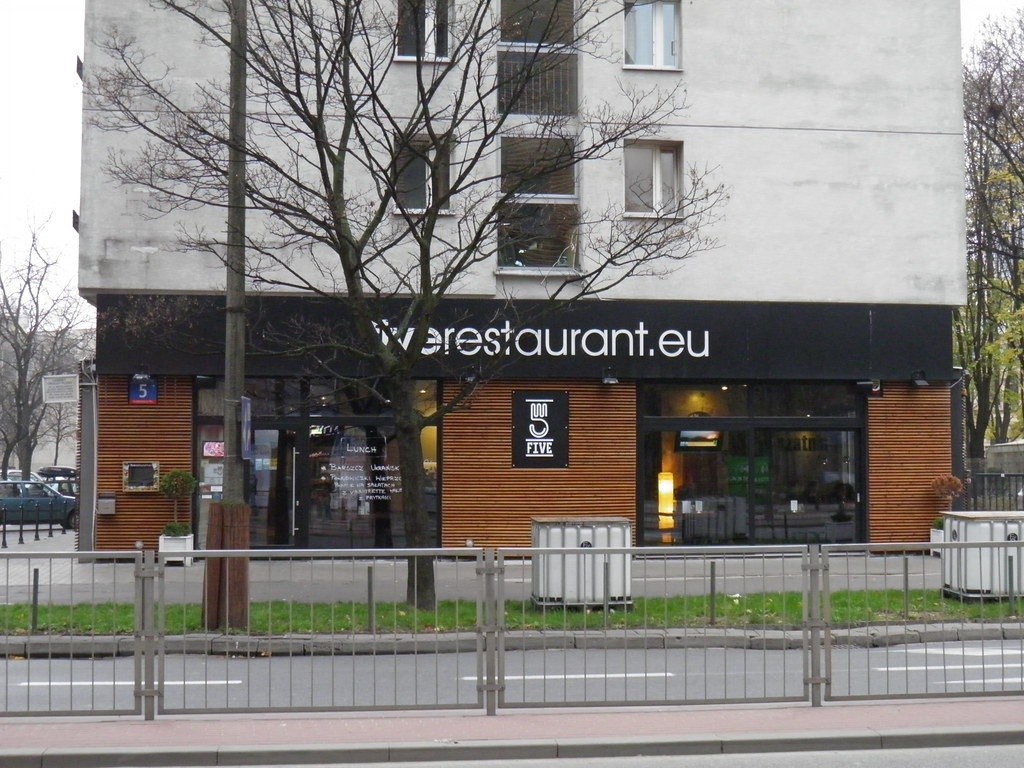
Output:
[158, 467, 196, 565]
[928, 474, 964, 555]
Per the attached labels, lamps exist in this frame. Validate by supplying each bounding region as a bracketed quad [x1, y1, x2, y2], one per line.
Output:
[600, 367, 619, 385]
[911, 370, 930, 387]
[463, 365, 482, 381]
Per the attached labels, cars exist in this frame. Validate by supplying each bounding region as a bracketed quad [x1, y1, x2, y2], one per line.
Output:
[0, 463, 77, 496]
[0, 480, 77, 530]
